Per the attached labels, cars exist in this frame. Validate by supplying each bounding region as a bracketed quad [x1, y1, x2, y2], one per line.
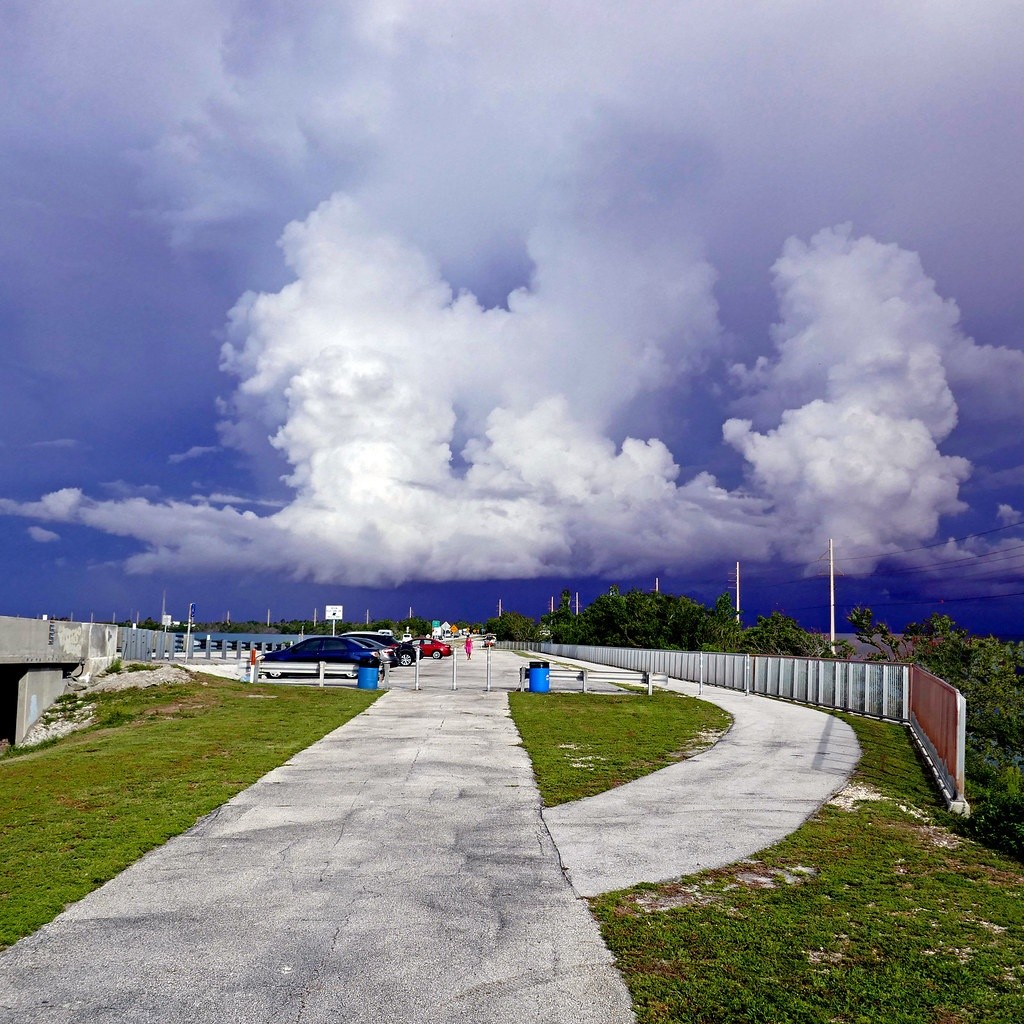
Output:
[341, 630, 425, 666]
[257, 635, 380, 680]
[343, 636, 400, 672]
[404, 637, 452, 660]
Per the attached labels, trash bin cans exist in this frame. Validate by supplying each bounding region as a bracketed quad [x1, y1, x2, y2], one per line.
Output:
[358, 656, 380, 689]
[529, 661, 550, 694]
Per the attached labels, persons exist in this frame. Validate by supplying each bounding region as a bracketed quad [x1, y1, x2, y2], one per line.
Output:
[464, 634, 473, 660]
